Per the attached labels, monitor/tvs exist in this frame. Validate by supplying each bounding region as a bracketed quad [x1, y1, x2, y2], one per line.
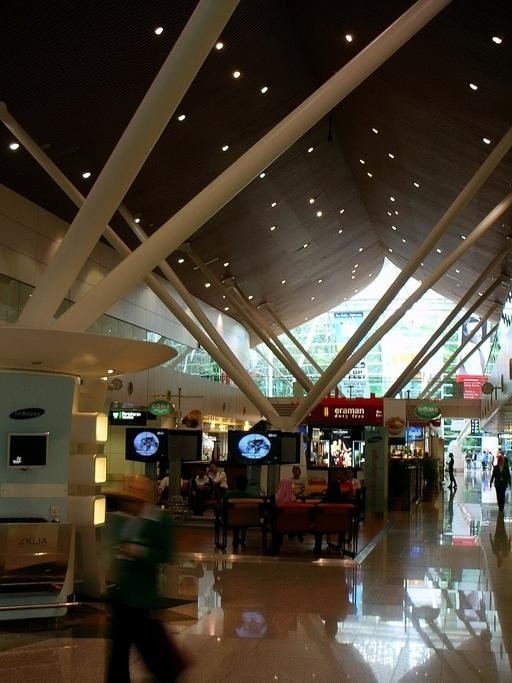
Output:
[282, 431, 301, 464]
[168, 429, 202, 462]
[404, 426, 425, 442]
[228, 430, 281, 465]
[125, 428, 168, 463]
[298, 424, 309, 438]
[7, 432, 51, 469]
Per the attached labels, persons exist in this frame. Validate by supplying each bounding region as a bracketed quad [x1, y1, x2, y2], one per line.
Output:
[335, 468, 354, 499]
[288, 464, 310, 496]
[203, 461, 227, 488]
[157, 474, 183, 495]
[193, 465, 211, 488]
[275, 478, 307, 543]
[322, 479, 350, 547]
[89, 473, 194, 682]
[446, 452, 457, 487]
[444, 487, 456, 527]
[488, 512, 510, 568]
[345, 468, 362, 491]
[460, 316, 487, 376]
[465, 446, 511, 512]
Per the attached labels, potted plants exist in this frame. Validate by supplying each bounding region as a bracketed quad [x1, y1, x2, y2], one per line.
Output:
[422, 457, 447, 501]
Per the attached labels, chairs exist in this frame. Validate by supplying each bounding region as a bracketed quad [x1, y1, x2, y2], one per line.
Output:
[214, 560, 358, 618]
[211, 499, 361, 558]
[203, 498, 219, 507]
